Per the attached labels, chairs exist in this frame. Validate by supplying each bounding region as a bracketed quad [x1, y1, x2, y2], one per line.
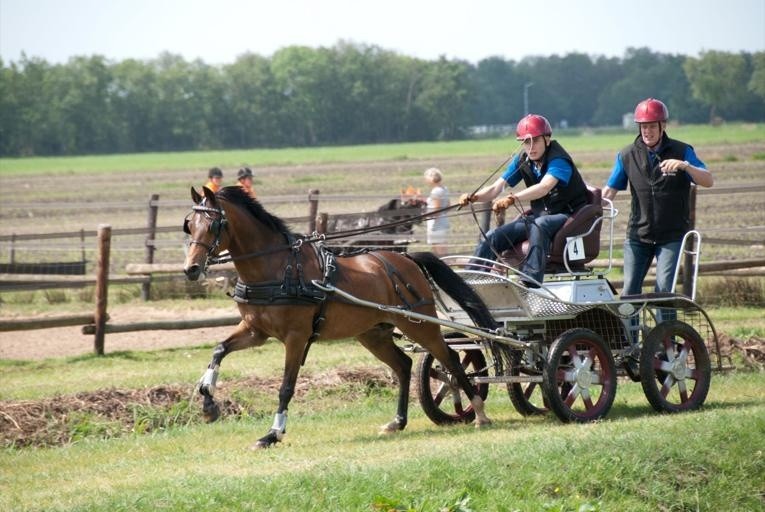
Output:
[496, 184, 603, 273]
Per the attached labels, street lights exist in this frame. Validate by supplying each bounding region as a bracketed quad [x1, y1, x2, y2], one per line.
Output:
[523, 82, 534, 116]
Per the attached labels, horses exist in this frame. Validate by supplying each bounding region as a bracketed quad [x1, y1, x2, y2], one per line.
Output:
[183, 186, 524, 452]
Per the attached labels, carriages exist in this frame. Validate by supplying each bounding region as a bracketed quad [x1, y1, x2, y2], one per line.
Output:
[182, 186, 736, 450]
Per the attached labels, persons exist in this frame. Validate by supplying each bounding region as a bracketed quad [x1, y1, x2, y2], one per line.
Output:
[600, 99, 715, 371]
[235, 168, 255, 198]
[422, 168, 452, 245]
[465, 113, 600, 289]
[198, 168, 222, 198]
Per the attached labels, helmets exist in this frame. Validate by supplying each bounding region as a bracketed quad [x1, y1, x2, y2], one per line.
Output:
[515, 113, 552, 141]
[633, 97, 669, 123]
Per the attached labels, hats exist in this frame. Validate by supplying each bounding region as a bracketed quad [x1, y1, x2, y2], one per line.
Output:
[236, 168, 254, 180]
[208, 167, 223, 178]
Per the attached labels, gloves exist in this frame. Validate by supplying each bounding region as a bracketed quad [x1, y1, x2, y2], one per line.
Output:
[459, 193, 478, 206]
[493, 196, 514, 211]
